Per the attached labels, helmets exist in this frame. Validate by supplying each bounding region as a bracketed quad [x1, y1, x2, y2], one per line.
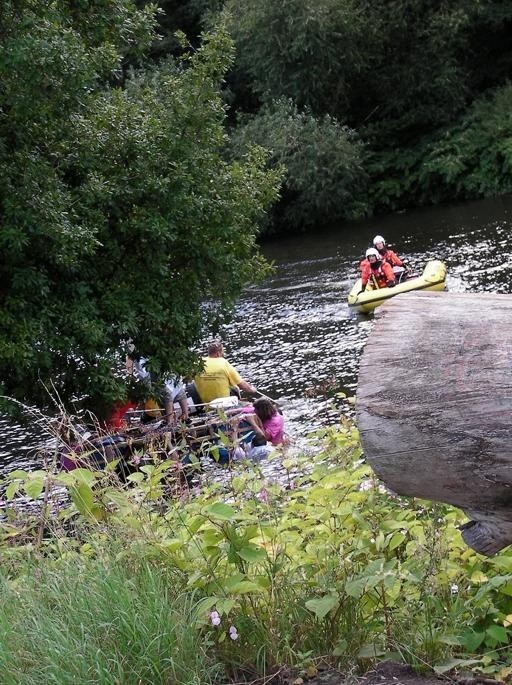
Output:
[373, 235, 385, 246]
[366, 248, 379, 261]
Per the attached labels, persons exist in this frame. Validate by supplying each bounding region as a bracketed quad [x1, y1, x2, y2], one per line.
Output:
[357, 248, 399, 293]
[60, 336, 285, 507]
[372, 234, 406, 268]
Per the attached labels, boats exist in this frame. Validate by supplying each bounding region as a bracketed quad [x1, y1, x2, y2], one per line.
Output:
[347, 260, 447, 313]
[59, 383, 266, 481]
